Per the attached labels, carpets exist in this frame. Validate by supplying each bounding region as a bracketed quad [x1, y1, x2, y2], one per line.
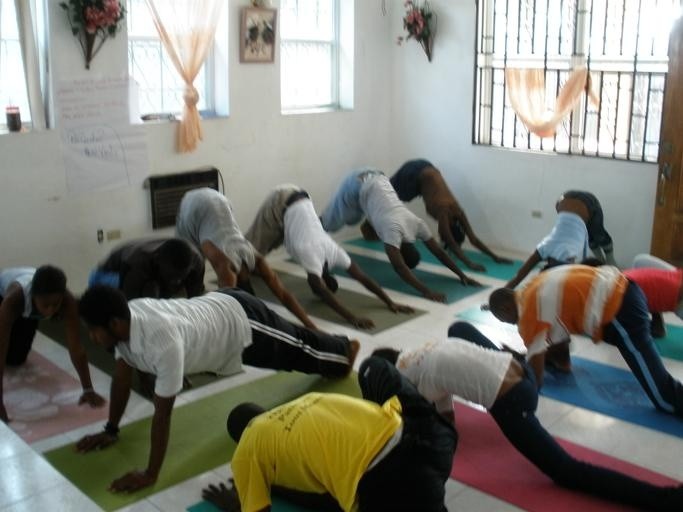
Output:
[186, 497, 306, 512]
[448, 398, 682, 511]
[647, 319, 683, 361]
[539, 354, 683, 439]
[286, 252, 493, 304]
[38, 319, 244, 405]
[43, 368, 362, 512]
[1, 349, 110, 443]
[344, 237, 527, 280]
[451, 304, 521, 337]
[210, 268, 427, 336]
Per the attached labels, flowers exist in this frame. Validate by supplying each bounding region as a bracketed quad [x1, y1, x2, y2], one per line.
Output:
[396, 0, 437, 62]
[59, 0, 128, 68]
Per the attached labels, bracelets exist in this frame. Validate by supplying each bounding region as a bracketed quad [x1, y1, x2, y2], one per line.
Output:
[107, 423, 120, 434]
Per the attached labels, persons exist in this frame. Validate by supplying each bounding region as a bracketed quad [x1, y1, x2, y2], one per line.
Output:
[75, 285, 359, 495]
[490, 262, 683, 417]
[243, 185, 415, 331]
[582, 254, 683, 337]
[88, 238, 205, 399]
[319, 168, 479, 304]
[201, 355, 456, 512]
[361, 160, 514, 272]
[373, 321, 683, 512]
[0, 265, 106, 423]
[481, 191, 618, 310]
[174, 187, 319, 331]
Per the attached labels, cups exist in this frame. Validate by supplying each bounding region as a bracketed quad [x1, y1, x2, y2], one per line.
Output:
[5, 106, 21, 133]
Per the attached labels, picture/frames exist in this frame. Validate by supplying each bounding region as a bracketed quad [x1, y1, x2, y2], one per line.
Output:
[240, 7, 277, 63]
